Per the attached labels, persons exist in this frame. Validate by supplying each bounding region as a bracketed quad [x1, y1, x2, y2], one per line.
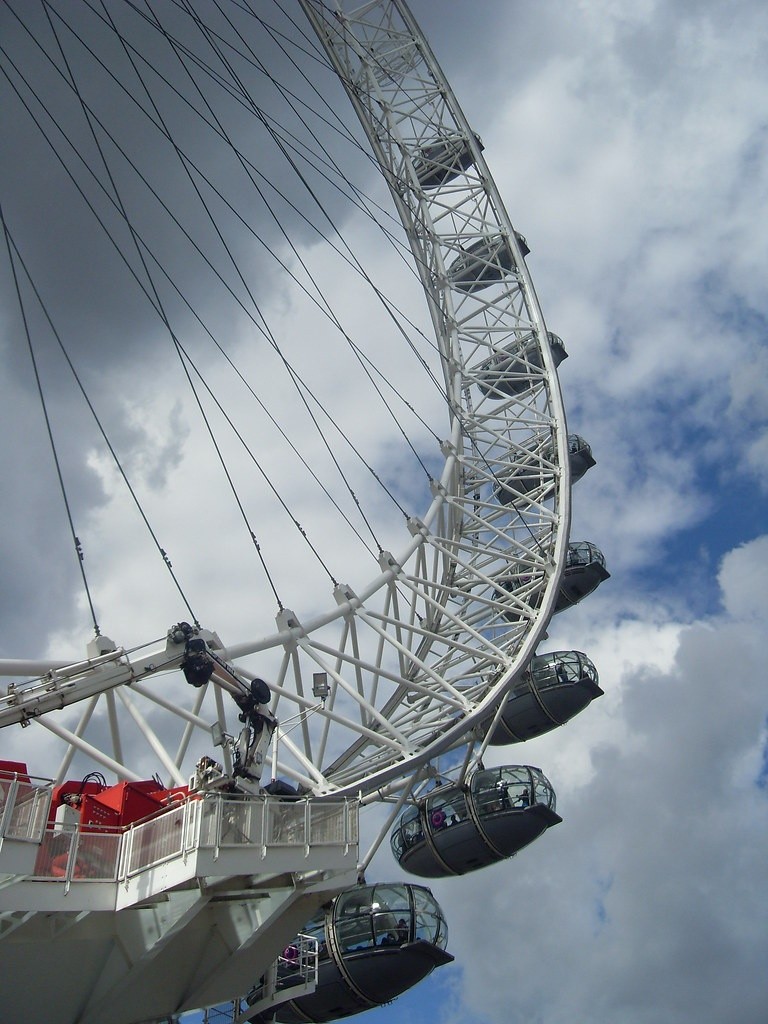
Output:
[573, 549, 580, 564]
[398, 781, 529, 853]
[571, 443, 577, 452]
[577, 668, 589, 680]
[318, 918, 422, 960]
[557, 663, 568, 682]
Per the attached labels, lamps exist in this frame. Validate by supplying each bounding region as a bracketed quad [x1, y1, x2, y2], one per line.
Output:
[278, 670, 328, 741]
[210, 721, 241, 765]
